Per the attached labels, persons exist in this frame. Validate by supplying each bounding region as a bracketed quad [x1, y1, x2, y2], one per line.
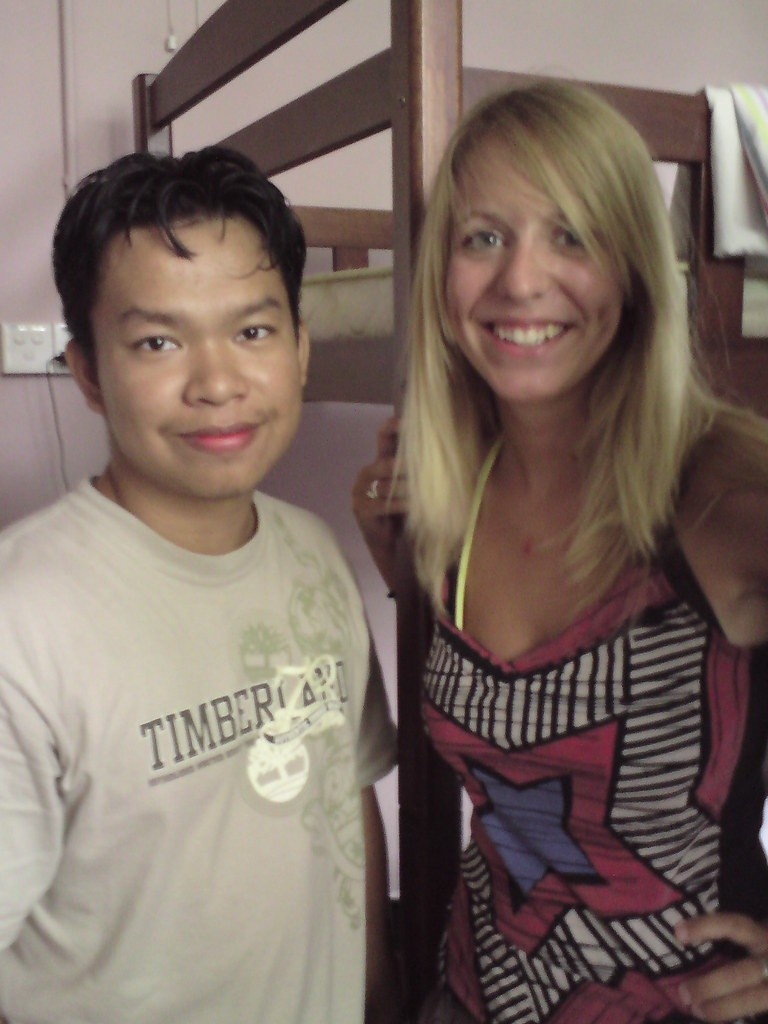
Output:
[0, 144, 399, 1024]
[350, 81, 768, 1024]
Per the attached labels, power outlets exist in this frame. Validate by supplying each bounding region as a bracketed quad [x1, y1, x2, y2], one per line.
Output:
[50, 323, 73, 375]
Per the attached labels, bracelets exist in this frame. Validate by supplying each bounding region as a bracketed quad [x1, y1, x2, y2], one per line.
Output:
[387, 588, 398, 598]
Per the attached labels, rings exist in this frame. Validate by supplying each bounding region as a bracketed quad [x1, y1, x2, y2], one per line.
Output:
[760, 958, 768, 981]
[367, 479, 379, 498]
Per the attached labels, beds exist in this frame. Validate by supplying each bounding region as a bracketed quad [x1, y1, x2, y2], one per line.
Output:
[134, 0, 768, 1024]
